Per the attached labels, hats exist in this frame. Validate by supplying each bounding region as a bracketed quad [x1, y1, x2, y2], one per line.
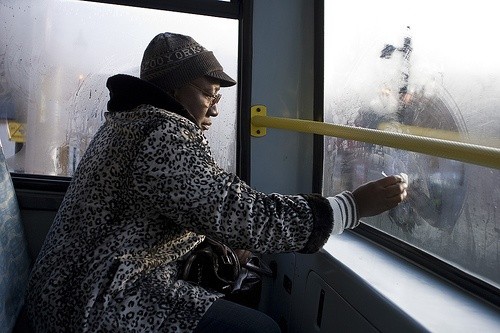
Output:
[140, 31, 236, 95]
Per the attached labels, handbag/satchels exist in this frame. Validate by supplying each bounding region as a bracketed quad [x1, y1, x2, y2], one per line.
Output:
[173, 233, 242, 296]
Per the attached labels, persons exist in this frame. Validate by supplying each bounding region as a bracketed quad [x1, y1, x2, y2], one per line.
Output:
[21, 32, 411, 333]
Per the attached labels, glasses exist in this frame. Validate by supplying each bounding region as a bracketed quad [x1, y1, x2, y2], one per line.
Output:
[185, 80, 222, 108]
